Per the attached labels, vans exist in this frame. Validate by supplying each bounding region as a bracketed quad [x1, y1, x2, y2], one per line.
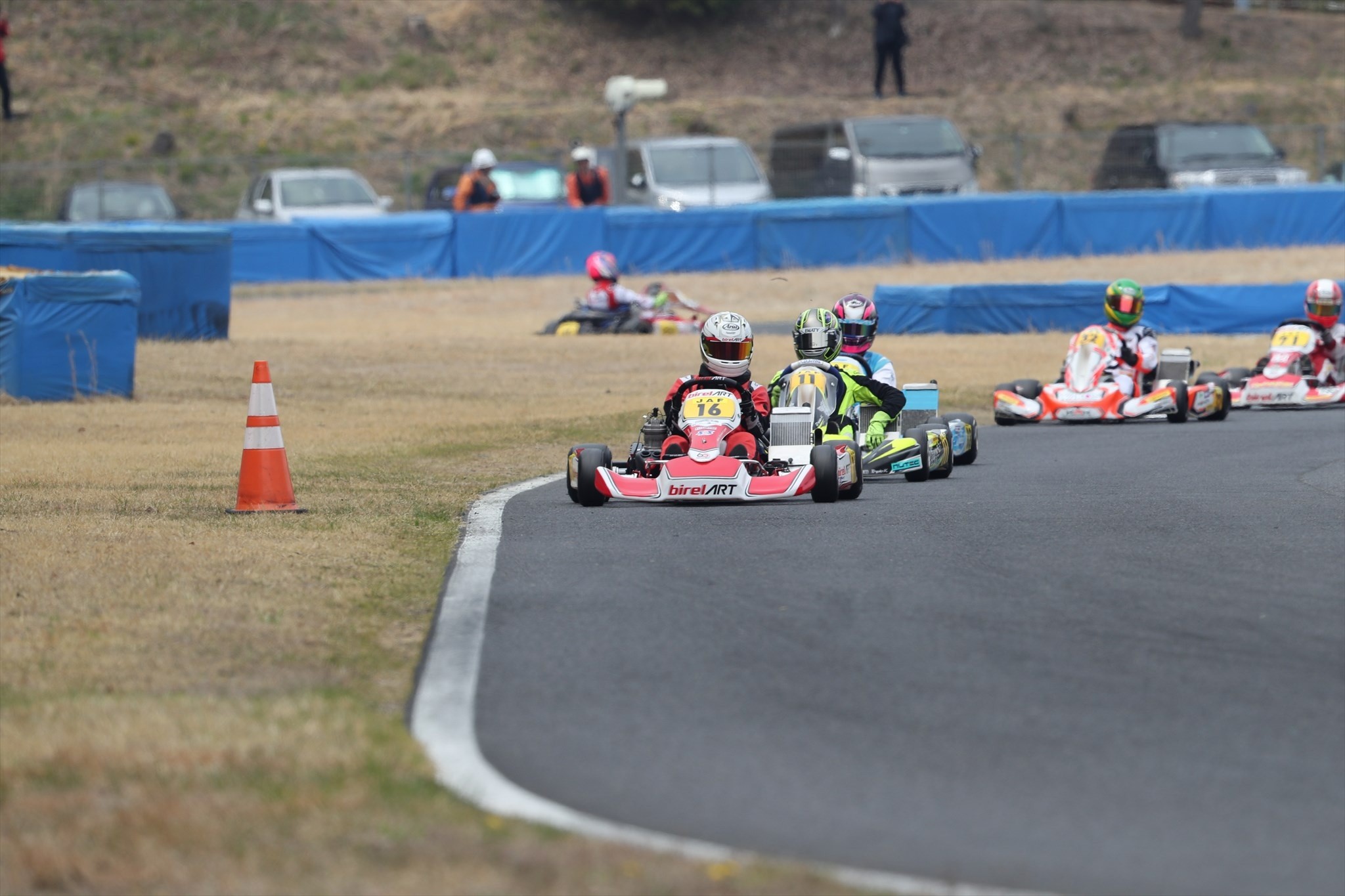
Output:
[596, 135, 774, 210]
[766, 114, 983, 199]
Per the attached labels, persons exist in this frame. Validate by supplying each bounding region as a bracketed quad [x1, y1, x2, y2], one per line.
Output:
[452, 149, 503, 212]
[1257, 278, 1345, 387]
[567, 146, 611, 208]
[870, 0, 906, 99]
[586, 250, 667, 318]
[1056, 278, 1159, 398]
[660, 311, 773, 478]
[771, 294, 906, 451]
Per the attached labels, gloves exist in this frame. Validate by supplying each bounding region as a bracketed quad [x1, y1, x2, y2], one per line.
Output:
[1320, 331, 1334, 345]
[1120, 345, 1138, 368]
[739, 388, 759, 430]
[770, 372, 781, 399]
[865, 421, 884, 450]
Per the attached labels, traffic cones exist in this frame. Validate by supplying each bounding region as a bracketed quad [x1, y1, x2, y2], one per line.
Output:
[223, 360, 308, 514]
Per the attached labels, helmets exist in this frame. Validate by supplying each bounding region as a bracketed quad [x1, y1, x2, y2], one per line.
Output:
[586, 250, 617, 282]
[699, 311, 754, 377]
[1305, 279, 1342, 329]
[471, 148, 497, 170]
[792, 308, 843, 363]
[571, 147, 598, 168]
[832, 293, 878, 353]
[1104, 278, 1144, 327]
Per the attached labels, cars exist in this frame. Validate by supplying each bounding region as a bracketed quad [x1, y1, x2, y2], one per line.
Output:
[56, 179, 192, 222]
[233, 168, 394, 220]
[422, 162, 569, 209]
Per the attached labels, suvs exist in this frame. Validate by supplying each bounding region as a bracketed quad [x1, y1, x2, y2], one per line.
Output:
[1094, 124, 1307, 188]
